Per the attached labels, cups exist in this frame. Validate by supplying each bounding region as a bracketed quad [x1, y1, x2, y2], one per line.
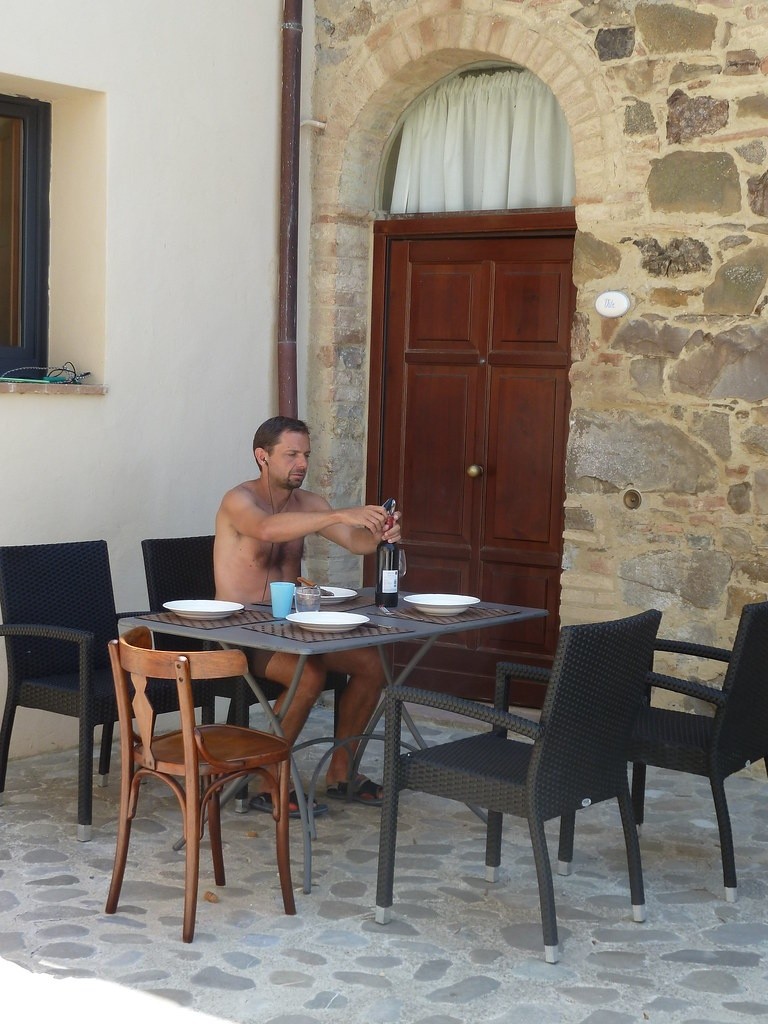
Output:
[296, 586, 321, 613]
[270, 582, 295, 618]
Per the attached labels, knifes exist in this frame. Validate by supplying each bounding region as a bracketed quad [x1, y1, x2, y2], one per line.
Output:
[298, 577, 333, 596]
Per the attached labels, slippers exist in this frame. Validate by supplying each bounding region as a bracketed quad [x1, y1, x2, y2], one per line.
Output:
[250, 789, 329, 819]
[326, 774, 383, 806]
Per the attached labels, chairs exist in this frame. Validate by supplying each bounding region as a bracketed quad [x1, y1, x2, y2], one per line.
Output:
[374, 608, 665, 966]
[0, 539, 219, 825]
[142, 536, 348, 814]
[106, 626, 297, 942]
[627, 602, 768, 904]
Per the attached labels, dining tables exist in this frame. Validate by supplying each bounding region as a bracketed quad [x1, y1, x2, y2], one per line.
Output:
[134, 587, 548, 895]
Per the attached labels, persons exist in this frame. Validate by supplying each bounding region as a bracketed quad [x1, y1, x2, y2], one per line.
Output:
[213, 415, 402, 818]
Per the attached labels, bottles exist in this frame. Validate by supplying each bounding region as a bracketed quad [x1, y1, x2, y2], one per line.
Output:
[375, 516, 399, 607]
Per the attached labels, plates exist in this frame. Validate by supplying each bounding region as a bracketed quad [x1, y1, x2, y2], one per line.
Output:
[162, 600, 244, 620]
[294, 587, 357, 604]
[404, 594, 480, 616]
[286, 612, 370, 633]
